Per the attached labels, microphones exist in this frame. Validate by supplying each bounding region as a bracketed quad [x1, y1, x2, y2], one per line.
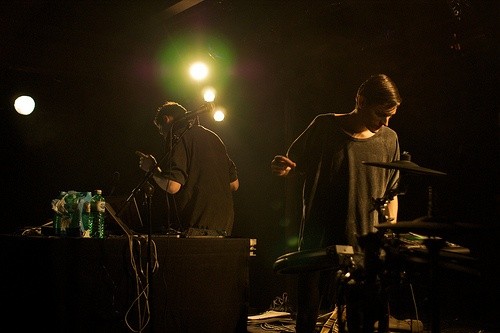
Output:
[175, 103, 213, 125]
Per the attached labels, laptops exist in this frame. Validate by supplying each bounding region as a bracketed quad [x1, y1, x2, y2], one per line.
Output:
[104, 203, 180, 238]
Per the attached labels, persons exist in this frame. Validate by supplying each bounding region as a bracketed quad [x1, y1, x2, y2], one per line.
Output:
[136, 103, 240, 238]
[272, 74, 401, 333]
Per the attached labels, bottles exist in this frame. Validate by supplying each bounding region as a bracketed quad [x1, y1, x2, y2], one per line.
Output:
[53, 190, 92, 238]
[90, 190, 106, 239]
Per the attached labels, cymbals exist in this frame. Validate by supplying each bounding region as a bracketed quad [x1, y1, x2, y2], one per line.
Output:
[361, 159, 446, 175]
